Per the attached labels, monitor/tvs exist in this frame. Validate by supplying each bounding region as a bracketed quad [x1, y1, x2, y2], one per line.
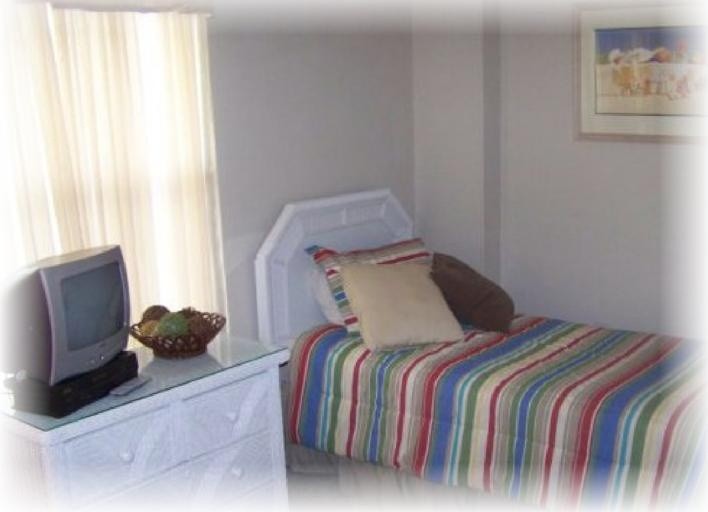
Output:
[2, 244, 130, 386]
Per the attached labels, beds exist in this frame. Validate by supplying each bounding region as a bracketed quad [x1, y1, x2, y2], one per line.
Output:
[250, 186, 706, 505]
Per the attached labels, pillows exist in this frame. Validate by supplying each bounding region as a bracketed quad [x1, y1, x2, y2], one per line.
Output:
[300, 237, 429, 334]
[338, 260, 464, 351]
[429, 253, 515, 335]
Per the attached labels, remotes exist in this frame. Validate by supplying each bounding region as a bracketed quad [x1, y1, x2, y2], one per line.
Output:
[110, 374, 150, 396]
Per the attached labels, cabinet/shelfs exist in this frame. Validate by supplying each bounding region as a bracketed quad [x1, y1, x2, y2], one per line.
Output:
[10, 337, 293, 504]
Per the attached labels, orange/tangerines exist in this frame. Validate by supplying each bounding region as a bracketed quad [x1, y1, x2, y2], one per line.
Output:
[158, 312, 189, 336]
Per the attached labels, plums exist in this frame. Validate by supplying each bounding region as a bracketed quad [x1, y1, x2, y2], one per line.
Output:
[142, 305, 168, 323]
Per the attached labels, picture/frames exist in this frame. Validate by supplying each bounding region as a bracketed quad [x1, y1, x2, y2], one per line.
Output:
[579, 6, 703, 140]
